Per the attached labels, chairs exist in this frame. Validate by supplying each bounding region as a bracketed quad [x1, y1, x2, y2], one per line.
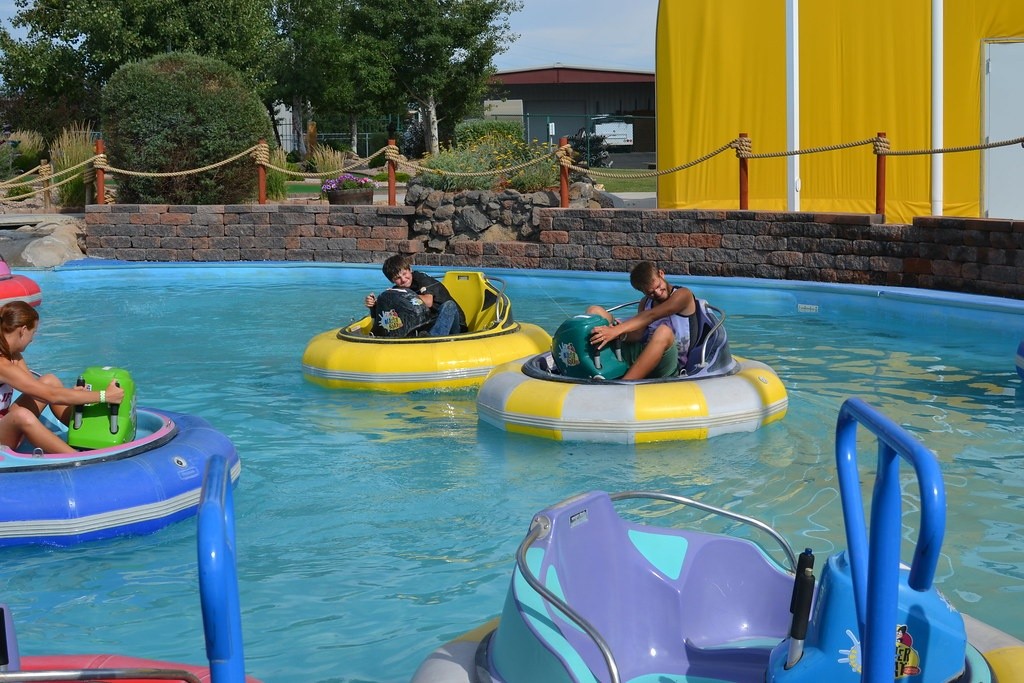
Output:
[679, 536, 817, 653]
[541, 491, 773, 683]
[438, 270, 503, 331]
[682, 295, 720, 374]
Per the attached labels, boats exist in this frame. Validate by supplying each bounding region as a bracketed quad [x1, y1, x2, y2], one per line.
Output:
[1, 456, 261, 683]
[403, 398, 1024, 683]
[297, 270, 557, 394]
[1, 366, 240, 543]
[474, 301, 792, 443]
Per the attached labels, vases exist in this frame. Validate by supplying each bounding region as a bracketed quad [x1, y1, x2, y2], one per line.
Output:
[325, 187, 374, 206]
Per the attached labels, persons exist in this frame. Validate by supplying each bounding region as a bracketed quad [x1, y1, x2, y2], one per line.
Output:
[0, 301, 124, 455]
[586, 262, 699, 380]
[365, 255, 468, 336]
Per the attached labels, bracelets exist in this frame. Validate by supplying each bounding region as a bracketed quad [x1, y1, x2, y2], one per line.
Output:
[100, 390, 106, 403]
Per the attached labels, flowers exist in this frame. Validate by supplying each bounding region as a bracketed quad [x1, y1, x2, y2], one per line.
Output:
[320, 172, 383, 200]
